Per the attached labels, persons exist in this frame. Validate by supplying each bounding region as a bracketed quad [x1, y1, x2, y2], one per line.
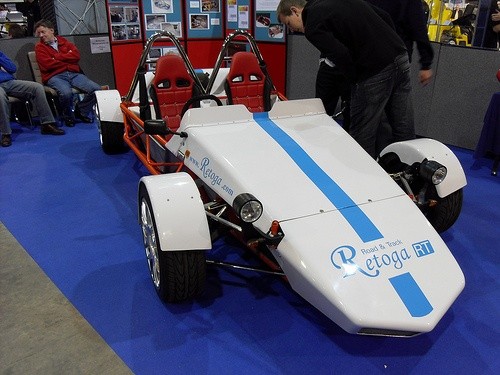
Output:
[34, 19, 101, 126]
[484, 0, 500, 47]
[276, 0, 435, 161]
[0, 51, 66, 147]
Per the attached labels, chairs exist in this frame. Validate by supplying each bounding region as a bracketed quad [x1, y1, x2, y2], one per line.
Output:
[6, 50, 96, 125]
[225, 51, 272, 112]
[150, 55, 195, 143]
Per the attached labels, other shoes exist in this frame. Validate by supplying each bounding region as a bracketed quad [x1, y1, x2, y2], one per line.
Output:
[64, 115, 75, 127]
[73, 109, 92, 123]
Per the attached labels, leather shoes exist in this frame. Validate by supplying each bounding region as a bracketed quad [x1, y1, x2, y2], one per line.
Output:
[0, 134, 12, 147]
[41, 123, 64, 135]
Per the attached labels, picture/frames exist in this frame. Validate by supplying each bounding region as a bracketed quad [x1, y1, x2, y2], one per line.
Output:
[108, 3, 142, 44]
